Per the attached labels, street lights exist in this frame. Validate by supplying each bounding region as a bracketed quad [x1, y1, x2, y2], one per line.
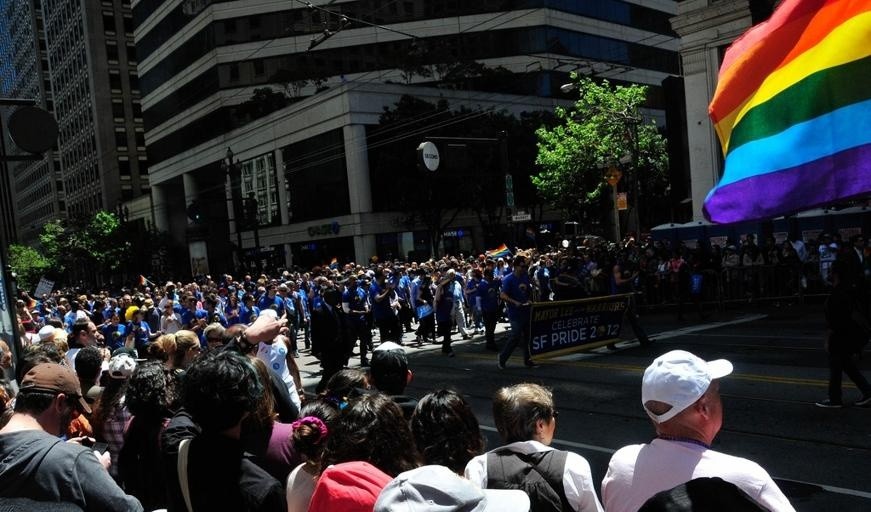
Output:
[111, 203, 130, 282]
[558, 80, 641, 243]
[218, 147, 247, 276]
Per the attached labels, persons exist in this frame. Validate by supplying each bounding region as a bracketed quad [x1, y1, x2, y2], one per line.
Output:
[1, 226, 871, 511]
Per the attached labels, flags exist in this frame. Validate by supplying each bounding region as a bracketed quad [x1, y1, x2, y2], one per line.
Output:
[700, 1, 871, 224]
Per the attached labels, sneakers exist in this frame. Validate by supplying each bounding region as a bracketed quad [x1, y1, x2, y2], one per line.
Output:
[607, 344, 620, 351]
[404, 326, 502, 357]
[815, 398, 843, 409]
[854, 385, 870, 407]
[524, 361, 542, 368]
[641, 338, 657, 348]
[496, 352, 506, 371]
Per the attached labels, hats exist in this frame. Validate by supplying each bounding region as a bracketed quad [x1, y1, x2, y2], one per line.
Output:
[727, 244, 736, 250]
[277, 279, 295, 292]
[640, 348, 735, 424]
[107, 353, 138, 379]
[111, 346, 148, 362]
[38, 323, 59, 339]
[20, 362, 94, 416]
[369, 340, 409, 379]
[497, 257, 504, 262]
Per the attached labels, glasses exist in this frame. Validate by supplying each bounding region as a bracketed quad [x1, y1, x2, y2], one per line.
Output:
[207, 337, 223, 343]
